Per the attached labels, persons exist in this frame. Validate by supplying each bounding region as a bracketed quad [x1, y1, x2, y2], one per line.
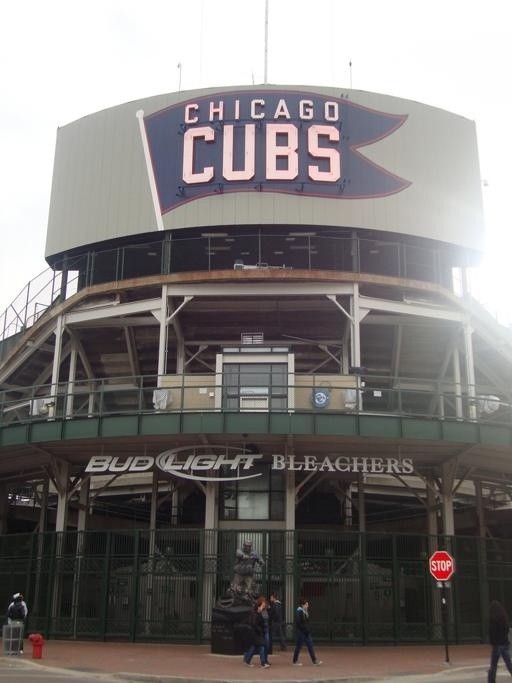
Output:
[7, 592, 29, 655]
[226, 538, 266, 600]
[257, 596, 272, 665]
[291, 599, 324, 666]
[270, 590, 288, 651]
[243, 598, 270, 668]
[487, 599, 512, 683]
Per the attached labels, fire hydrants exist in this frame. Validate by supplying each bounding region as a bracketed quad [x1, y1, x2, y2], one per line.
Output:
[29, 633, 46, 658]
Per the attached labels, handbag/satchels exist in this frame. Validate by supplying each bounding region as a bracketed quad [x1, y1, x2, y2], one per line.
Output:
[253, 636, 265, 645]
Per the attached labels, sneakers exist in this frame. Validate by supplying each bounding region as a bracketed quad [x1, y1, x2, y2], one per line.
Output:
[245, 662, 270, 667]
[293, 660, 322, 666]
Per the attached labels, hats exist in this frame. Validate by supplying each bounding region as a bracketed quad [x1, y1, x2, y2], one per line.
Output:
[13, 593, 20, 599]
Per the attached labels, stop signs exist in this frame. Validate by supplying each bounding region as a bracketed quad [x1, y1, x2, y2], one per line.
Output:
[429, 550, 454, 581]
